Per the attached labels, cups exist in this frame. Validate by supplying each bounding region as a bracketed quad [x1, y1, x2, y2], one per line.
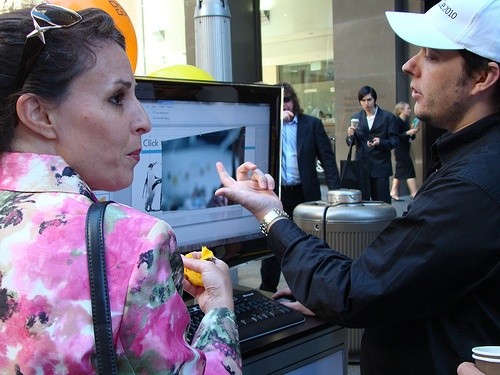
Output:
[472, 346, 500, 375]
[351, 119, 359, 128]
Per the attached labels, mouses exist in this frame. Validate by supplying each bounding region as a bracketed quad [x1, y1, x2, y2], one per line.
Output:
[274, 295, 297, 303]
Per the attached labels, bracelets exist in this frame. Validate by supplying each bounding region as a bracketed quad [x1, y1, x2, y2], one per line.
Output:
[258, 208, 290, 236]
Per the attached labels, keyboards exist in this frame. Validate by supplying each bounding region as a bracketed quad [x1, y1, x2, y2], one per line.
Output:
[184, 290, 305, 347]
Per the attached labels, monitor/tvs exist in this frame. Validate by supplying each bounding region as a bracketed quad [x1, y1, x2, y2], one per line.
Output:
[90, 76, 284, 269]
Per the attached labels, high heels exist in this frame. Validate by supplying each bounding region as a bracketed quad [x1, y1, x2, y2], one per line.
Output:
[390, 195, 404, 201]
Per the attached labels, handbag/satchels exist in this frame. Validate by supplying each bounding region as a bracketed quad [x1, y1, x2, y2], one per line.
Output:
[340, 136, 360, 190]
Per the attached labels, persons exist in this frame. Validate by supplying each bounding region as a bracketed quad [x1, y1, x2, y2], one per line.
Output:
[457, 361, 486, 375]
[345, 85, 402, 205]
[390, 102, 420, 202]
[215, 0, 500, 375]
[258, 83, 342, 293]
[0, 3, 243, 375]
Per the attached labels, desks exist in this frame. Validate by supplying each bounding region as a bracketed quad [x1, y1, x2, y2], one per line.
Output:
[181, 284, 349, 375]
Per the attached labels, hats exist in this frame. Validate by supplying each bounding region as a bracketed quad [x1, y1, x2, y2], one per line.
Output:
[385, 0, 500, 63]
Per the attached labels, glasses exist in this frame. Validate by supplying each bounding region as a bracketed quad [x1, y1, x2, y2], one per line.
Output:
[14, 2, 82, 128]
[284, 95, 293, 103]
